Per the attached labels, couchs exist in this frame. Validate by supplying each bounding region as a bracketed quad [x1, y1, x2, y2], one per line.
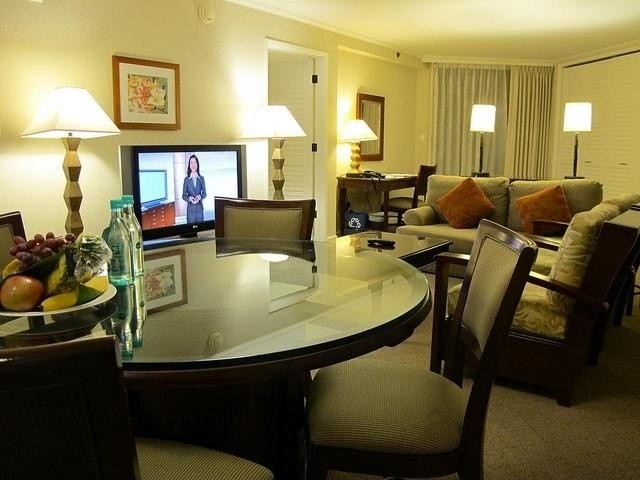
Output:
[429, 190, 640, 408]
[394, 174, 604, 279]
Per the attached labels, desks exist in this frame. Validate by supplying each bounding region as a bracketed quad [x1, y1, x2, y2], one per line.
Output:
[336, 173, 418, 236]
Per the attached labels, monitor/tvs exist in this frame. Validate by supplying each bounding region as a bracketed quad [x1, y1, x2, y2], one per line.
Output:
[119, 144, 247, 251]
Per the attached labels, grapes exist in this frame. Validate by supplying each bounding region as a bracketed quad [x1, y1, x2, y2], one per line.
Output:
[9, 232, 75, 271]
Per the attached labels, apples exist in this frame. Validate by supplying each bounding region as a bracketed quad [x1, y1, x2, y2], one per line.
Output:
[0, 273, 45, 312]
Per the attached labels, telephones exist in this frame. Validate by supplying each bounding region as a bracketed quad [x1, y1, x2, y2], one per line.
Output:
[364, 171, 385, 179]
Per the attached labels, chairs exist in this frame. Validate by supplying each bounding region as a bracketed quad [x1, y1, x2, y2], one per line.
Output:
[303, 219, 539, 480]
[0, 332, 275, 480]
[213, 196, 315, 241]
[1, 211, 26, 278]
[382, 165, 438, 226]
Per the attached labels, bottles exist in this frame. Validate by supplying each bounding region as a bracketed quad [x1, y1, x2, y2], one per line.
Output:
[110, 275, 145, 359]
[104, 193, 144, 287]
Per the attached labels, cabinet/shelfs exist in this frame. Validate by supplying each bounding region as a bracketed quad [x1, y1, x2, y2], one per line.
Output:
[142, 201, 175, 230]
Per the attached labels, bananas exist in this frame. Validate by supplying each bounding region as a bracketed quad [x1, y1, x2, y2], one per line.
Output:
[36, 276, 109, 312]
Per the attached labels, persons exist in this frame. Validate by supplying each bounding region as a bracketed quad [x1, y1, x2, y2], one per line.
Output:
[181, 154, 207, 222]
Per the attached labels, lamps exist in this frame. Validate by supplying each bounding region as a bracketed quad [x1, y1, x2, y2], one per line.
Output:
[469, 104, 496, 177]
[20, 87, 122, 237]
[563, 102, 592, 179]
[337, 120, 380, 176]
[239, 106, 308, 200]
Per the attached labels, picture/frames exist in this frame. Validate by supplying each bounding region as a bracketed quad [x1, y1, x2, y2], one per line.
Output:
[143, 248, 188, 316]
[112, 55, 181, 130]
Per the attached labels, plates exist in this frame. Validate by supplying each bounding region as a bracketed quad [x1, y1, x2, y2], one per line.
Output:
[0, 301, 119, 336]
[0, 280, 118, 318]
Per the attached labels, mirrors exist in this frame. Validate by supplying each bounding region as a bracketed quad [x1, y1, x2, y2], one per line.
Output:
[356, 91, 385, 162]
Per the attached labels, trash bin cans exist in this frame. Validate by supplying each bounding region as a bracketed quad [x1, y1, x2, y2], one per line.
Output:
[343, 212, 369, 236]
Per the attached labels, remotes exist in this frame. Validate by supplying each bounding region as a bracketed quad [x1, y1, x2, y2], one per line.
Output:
[368, 238, 395, 246]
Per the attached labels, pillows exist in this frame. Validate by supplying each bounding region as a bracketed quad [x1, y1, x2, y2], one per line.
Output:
[508, 178, 603, 233]
[600, 190, 639, 211]
[516, 185, 572, 234]
[435, 176, 496, 229]
[548, 203, 621, 311]
[425, 173, 509, 230]
